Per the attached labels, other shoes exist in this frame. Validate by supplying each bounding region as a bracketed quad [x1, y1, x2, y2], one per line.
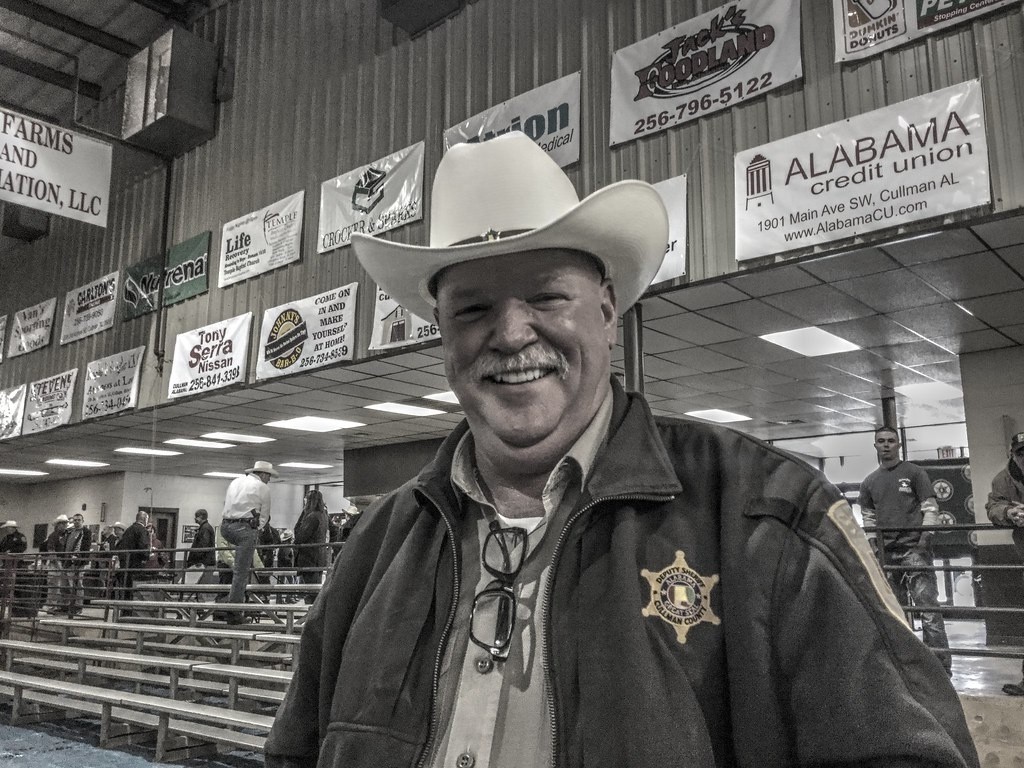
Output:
[1001, 678, 1024, 696]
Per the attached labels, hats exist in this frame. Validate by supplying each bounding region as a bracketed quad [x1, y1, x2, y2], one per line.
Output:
[244, 461, 279, 478]
[108, 522, 127, 529]
[1011, 432, 1024, 452]
[342, 506, 360, 517]
[57, 514, 69, 522]
[280, 529, 294, 541]
[0, 520, 17, 528]
[349, 130, 670, 326]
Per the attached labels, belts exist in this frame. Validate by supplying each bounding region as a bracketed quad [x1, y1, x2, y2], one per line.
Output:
[223, 518, 250, 522]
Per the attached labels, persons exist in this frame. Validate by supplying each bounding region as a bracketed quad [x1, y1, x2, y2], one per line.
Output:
[0, 511, 170, 616]
[985, 432, 1024, 695]
[264, 131, 981, 768]
[176, 460, 361, 620]
[857, 427, 953, 680]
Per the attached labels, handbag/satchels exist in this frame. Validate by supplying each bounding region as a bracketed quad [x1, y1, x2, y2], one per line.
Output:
[62, 555, 73, 569]
[157, 551, 169, 566]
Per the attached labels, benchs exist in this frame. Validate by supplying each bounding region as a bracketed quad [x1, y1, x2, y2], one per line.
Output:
[0, 583, 325, 761]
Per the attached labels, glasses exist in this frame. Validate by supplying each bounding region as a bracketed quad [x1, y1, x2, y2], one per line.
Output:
[59, 522, 66, 526]
[467, 519, 528, 663]
